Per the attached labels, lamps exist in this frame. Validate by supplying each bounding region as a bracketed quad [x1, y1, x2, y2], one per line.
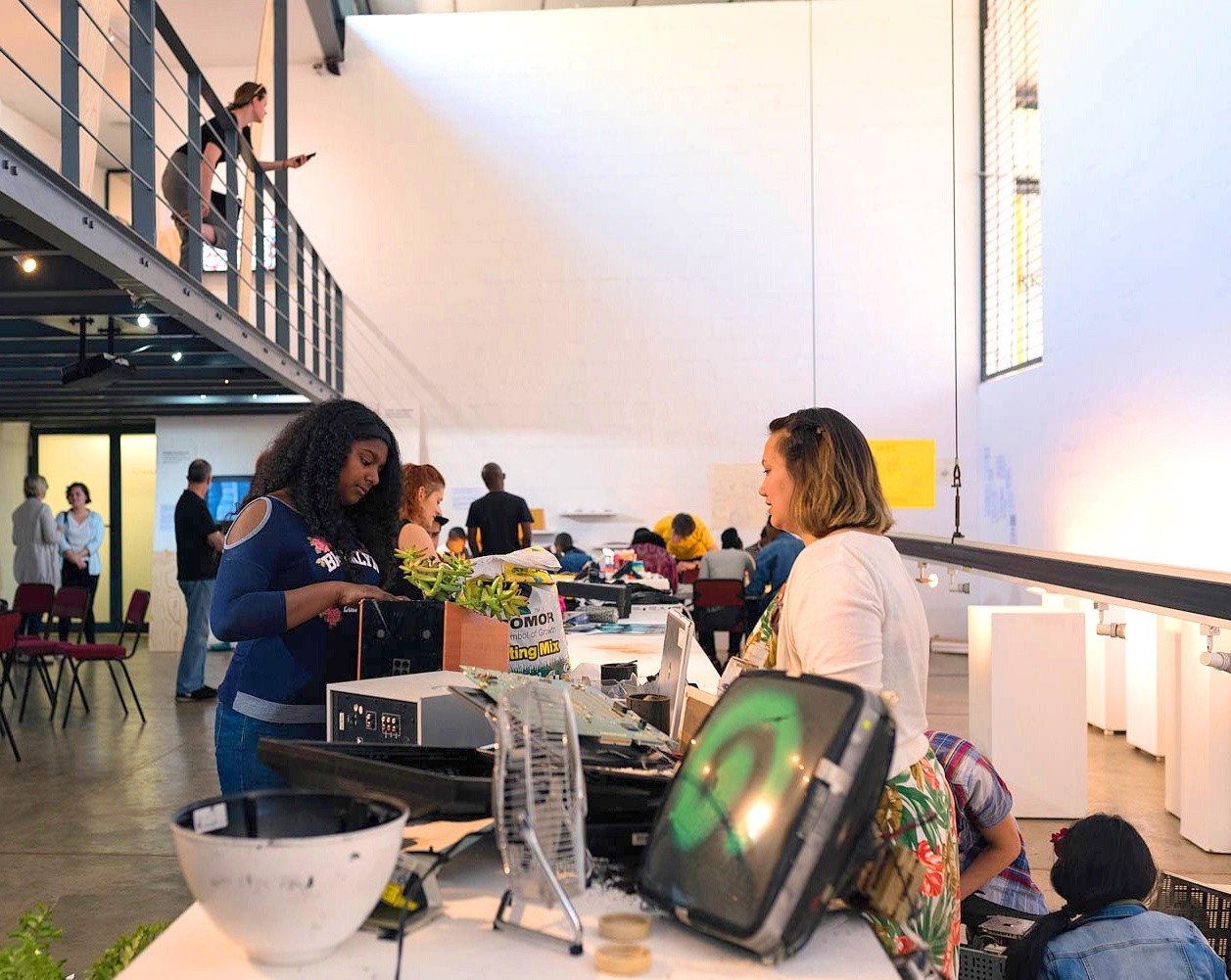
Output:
[915, 563, 938, 587]
[1199, 623, 1231, 673]
[1094, 602, 1127, 638]
[948, 568, 970, 594]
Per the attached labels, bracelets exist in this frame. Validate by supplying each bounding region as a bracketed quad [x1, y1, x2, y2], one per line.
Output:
[283, 158, 286, 170]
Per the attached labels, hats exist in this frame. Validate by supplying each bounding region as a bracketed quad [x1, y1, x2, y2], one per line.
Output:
[434, 509, 449, 525]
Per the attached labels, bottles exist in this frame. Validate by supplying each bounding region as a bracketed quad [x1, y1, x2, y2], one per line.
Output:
[563, 597, 576, 623]
[603, 548, 644, 579]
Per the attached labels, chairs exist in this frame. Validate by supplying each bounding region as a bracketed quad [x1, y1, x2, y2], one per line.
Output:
[0, 581, 151, 760]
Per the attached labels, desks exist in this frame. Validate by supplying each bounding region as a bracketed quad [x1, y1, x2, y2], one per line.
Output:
[98, 599, 898, 980]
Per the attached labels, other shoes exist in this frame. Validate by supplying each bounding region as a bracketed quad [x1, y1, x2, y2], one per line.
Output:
[18, 655, 54, 666]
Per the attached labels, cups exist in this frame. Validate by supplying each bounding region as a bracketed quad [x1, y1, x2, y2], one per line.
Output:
[601, 663, 638, 685]
[626, 693, 671, 737]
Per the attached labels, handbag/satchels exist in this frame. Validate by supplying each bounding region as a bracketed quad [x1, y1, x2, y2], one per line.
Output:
[439, 545, 571, 681]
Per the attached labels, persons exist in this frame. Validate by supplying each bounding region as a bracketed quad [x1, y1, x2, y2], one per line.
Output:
[55, 483, 104, 658]
[389, 461, 442, 599]
[725, 407, 960, 980]
[426, 462, 594, 570]
[11, 474, 64, 637]
[1008, 813, 1227, 980]
[209, 398, 407, 795]
[174, 460, 225, 701]
[631, 513, 806, 677]
[162, 81, 308, 280]
[925, 726, 1048, 931]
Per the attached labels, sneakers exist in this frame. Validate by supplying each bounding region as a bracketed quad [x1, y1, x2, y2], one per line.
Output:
[175, 685, 217, 703]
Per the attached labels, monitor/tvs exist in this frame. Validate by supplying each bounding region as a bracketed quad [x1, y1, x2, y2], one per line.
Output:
[205, 475, 255, 529]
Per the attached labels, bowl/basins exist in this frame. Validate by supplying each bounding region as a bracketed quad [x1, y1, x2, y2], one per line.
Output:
[169, 788, 411, 969]
